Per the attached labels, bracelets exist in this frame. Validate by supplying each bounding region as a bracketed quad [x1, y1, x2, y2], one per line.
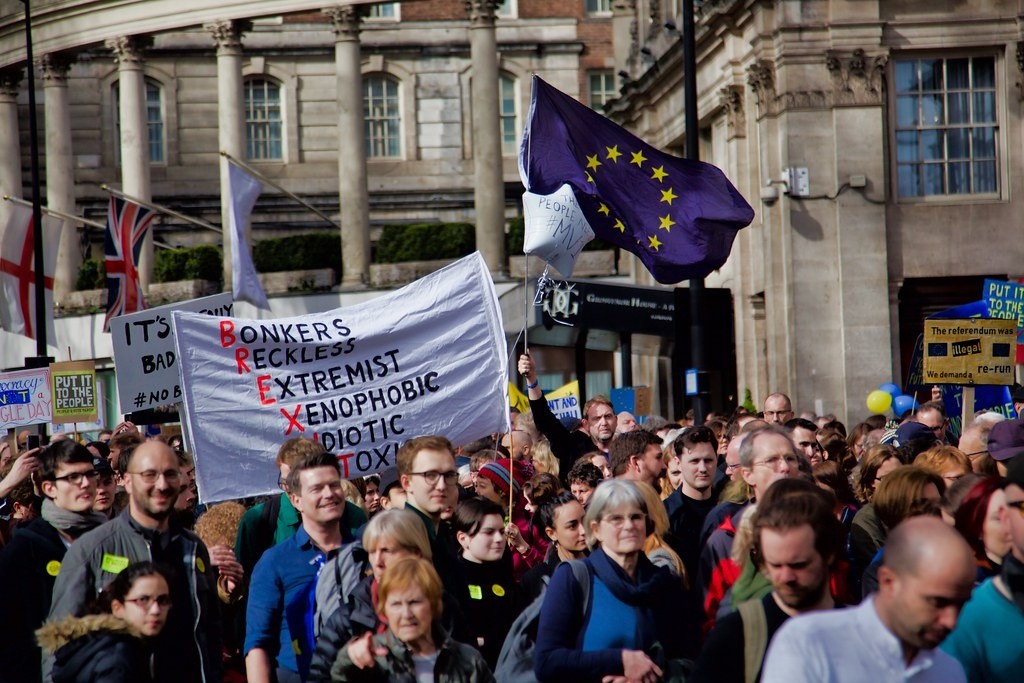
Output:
[526, 379, 538, 388]
[521, 549, 530, 556]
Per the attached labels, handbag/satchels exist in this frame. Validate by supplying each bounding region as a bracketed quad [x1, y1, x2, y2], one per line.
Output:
[492, 560, 591, 683]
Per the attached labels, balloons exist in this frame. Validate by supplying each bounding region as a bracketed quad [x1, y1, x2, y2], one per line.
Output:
[866, 391, 892, 414]
[893, 395, 920, 417]
[879, 383, 902, 405]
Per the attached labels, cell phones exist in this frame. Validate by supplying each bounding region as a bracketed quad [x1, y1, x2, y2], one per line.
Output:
[27, 435, 39, 451]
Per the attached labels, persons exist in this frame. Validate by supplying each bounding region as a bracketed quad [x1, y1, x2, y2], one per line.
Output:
[0, 350, 1024, 683]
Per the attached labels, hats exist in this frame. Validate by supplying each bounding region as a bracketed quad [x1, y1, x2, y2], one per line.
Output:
[379, 466, 400, 497]
[893, 422, 935, 448]
[987, 420, 1024, 461]
[477, 458, 522, 501]
[643, 416, 682, 434]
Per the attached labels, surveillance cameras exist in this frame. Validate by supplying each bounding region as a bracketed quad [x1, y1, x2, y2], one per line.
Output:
[761, 187, 779, 207]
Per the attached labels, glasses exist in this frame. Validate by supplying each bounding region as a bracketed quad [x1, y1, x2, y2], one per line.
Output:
[874, 475, 886, 482]
[728, 463, 739, 470]
[132, 470, 182, 483]
[125, 596, 174, 613]
[408, 470, 459, 486]
[278, 472, 290, 492]
[1006, 500, 1024, 512]
[931, 419, 945, 434]
[52, 470, 100, 485]
[764, 410, 791, 417]
[751, 454, 802, 469]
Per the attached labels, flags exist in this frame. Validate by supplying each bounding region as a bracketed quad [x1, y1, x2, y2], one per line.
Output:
[228, 161, 270, 310]
[102, 196, 158, 333]
[0, 200, 65, 349]
[518, 76, 754, 286]
